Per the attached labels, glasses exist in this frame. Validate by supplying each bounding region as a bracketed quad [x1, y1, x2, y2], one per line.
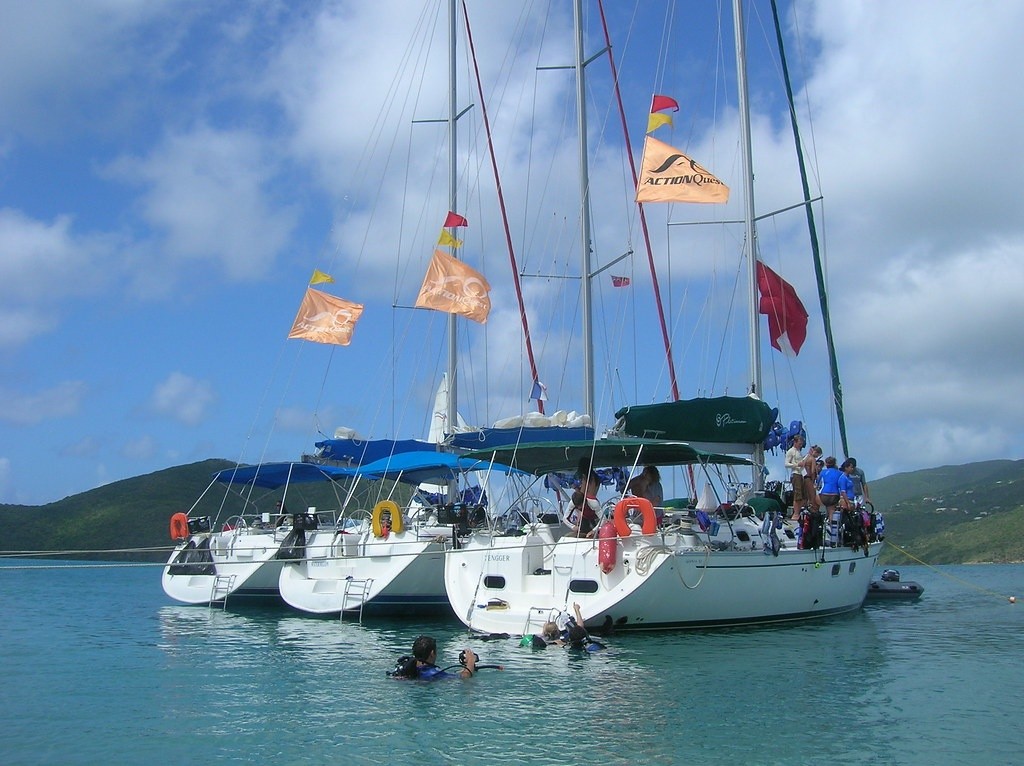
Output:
[277, 505, 282, 506]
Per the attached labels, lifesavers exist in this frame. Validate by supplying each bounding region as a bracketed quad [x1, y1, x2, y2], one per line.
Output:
[373, 500, 402, 536]
[171, 512, 188, 539]
[614, 498, 656, 537]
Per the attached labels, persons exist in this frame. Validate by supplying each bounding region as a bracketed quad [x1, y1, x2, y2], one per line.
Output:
[620, 466, 664, 528]
[446, 503, 490, 527]
[543, 601, 584, 645]
[277, 500, 288, 527]
[785, 435, 871, 522]
[562, 457, 606, 538]
[412, 635, 476, 680]
[569, 625, 607, 649]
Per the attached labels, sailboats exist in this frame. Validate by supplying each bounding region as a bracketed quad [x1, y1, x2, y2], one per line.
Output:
[445, 0, 882, 635]
[157, 1, 551, 605]
[277, 1, 682, 620]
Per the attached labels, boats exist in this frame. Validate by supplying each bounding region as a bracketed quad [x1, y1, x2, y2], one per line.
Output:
[868, 579, 925, 601]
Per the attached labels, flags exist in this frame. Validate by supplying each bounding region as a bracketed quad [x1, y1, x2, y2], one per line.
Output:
[416, 249, 492, 324]
[635, 136, 730, 203]
[612, 276, 630, 287]
[756, 259, 809, 361]
[288, 287, 364, 347]
[530, 382, 549, 401]
